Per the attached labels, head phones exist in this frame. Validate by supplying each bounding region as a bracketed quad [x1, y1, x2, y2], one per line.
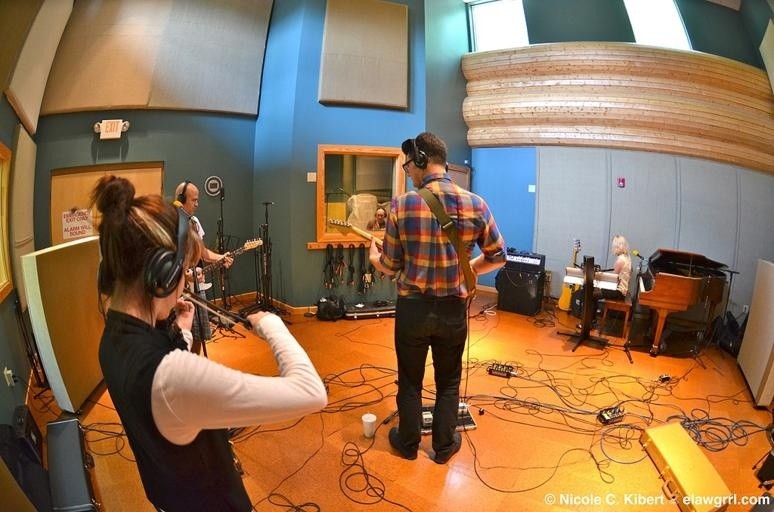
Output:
[413, 139, 449, 173]
[96, 205, 192, 297]
[177, 180, 195, 204]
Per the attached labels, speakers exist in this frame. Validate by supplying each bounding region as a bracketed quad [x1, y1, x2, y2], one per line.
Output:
[497, 267, 546, 316]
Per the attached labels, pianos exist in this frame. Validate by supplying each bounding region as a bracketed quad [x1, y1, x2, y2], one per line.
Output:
[637, 249, 729, 356]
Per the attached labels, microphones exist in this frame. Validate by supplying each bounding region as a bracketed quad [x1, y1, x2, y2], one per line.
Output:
[262, 202, 276, 205]
[633, 250, 644, 260]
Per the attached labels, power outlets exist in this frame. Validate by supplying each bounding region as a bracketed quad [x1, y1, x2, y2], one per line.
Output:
[2, 366, 14, 386]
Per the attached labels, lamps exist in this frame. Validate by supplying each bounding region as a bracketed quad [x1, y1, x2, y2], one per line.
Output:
[93, 119, 130, 140]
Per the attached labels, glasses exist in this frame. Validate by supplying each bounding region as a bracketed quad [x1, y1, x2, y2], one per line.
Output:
[402, 159, 414, 172]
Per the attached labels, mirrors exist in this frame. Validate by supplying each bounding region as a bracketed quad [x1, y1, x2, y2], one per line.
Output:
[311, 142, 408, 245]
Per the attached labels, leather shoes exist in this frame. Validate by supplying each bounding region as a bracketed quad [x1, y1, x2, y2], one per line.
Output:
[433, 431, 462, 465]
[389, 426, 420, 461]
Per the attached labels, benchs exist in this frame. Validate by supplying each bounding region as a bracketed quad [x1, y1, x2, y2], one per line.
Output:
[597, 289, 631, 345]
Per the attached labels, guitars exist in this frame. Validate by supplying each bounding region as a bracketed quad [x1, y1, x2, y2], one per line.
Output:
[187, 238, 263, 294]
[558, 240, 582, 312]
[327, 219, 384, 250]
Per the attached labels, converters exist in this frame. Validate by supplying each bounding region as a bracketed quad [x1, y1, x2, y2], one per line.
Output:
[598, 406, 625, 425]
[660, 374, 670, 383]
[490, 363, 513, 378]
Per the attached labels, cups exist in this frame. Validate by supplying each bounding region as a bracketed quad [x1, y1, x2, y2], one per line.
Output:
[361, 413, 375, 439]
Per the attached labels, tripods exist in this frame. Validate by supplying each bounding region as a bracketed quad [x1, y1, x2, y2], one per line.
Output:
[210, 215, 293, 338]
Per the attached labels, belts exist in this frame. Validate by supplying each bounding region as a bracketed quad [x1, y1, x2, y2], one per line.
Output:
[398, 295, 466, 305]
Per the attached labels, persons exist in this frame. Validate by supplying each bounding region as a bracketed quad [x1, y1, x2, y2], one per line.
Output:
[576, 235, 633, 330]
[91, 175, 328, 512]
[369, 131, 507, 465]
[175, 180, 234, 356]
[367, 207, 387, 231]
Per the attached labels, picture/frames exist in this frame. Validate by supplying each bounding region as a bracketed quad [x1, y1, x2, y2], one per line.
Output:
[446, 160, 472, 193]
